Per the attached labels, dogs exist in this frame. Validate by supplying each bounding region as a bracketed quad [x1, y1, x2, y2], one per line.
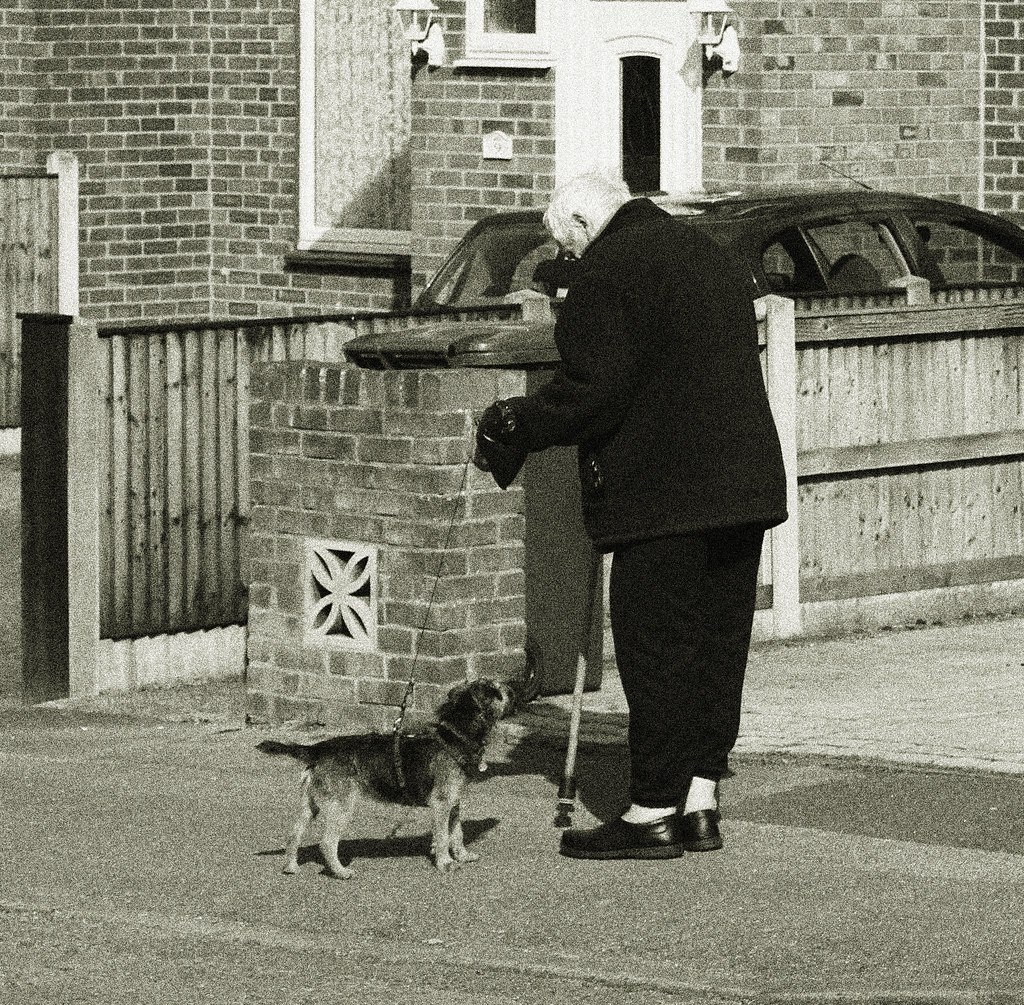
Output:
[255, 676, 519, 884]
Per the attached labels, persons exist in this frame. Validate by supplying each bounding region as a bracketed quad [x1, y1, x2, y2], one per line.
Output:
[478, 171, 789, 859]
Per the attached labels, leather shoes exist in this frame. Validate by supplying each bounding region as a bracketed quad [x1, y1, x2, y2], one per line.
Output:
[681, 808, 723, 853]
[559, 813, 686, 861]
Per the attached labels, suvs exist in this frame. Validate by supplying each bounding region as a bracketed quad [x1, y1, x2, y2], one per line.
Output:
[410, 185, 1024, 319]
